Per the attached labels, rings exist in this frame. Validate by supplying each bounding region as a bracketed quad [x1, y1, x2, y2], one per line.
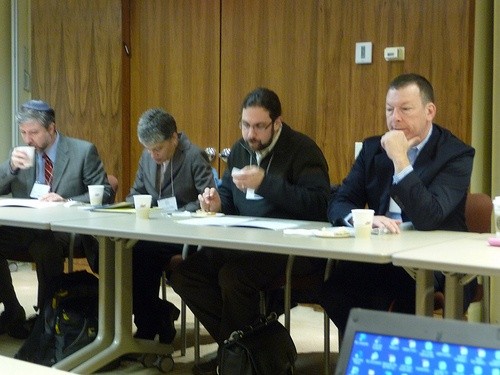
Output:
[242, 184, 244, 190]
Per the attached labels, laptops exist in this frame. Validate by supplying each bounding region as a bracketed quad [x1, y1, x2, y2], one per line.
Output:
[336, 308, 500, 375]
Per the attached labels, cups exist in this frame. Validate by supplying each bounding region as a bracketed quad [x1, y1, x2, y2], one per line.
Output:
[352, 209, 374, 243]
[133, 195, 153, 220]
[88, 186, 104, 207]
[18, 146, 35, 169]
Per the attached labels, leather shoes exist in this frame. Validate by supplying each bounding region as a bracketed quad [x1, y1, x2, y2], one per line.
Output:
[134, 299, 180, 344]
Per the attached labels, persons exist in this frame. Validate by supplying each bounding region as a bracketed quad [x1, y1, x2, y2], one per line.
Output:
[125, 86, 332, 375]
[317, 73, 476, 334]
[0, 97, 113, 332]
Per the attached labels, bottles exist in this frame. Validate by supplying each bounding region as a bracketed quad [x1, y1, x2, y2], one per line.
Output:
[490, 196, 500, 247]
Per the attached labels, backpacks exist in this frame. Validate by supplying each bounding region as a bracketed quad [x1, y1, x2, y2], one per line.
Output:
[32, 270, 121, 372]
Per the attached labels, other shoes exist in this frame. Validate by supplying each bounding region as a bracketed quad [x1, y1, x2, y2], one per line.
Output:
[9, 314, 40, 339]
[0, 307, 26, 331]
[192, 358, 216, 375]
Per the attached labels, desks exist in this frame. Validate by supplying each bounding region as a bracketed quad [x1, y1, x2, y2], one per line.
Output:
[0, 197, 500, 375]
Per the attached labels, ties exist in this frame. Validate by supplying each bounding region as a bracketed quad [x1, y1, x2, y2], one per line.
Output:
[43, 155, 54, 185]
[407, 148, 417, 165]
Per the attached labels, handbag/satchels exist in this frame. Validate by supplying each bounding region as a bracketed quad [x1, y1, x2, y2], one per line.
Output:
[217, 312, 297, 375]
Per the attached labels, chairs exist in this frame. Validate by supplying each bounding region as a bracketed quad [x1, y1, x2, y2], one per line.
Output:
[161, 243, 330, 375]
[68, 175, 119, 273]
[325, 193, 493, 375]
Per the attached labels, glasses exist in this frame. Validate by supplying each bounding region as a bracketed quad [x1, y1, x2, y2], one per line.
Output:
[238, 118, 272, 130]
